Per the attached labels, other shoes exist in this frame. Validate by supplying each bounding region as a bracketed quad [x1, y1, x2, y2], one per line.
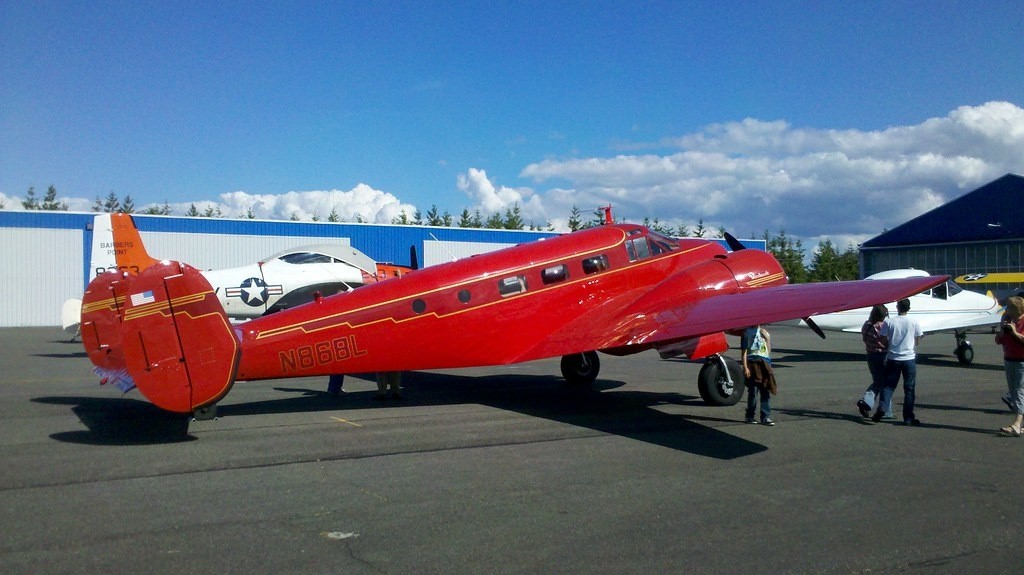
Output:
[858, 401, 869, 418]
[392, 393, 406, 401]
[328, 389, 351, 397]
[882, 415, 897, 419]
[379, 395, 389, 403]
[873, 409, 885, 423]
[906, 420, 919, 426]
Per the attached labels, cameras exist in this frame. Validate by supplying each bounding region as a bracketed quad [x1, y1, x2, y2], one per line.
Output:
[1003, 325, 1013, 333]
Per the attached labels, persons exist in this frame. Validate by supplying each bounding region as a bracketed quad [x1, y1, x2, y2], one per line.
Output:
[994, 291, 1024, 436]
[741, 325, 776, 425]
[871, 299, 923, 423]
[857, 305, 897, 419]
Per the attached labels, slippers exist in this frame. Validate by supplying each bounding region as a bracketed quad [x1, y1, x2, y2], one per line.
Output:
[999, 426, 1020, 437]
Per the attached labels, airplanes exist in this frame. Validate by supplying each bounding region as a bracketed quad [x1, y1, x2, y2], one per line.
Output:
[796, 266, 1005, 365]
[76, 206, 952, 422]
[61, 212, 417, 341]
[954, 273, 1024, 314]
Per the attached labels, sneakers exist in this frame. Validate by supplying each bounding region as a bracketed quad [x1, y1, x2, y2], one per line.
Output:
[764, 418, 775, 425]
[745, 417, 757, 424]
